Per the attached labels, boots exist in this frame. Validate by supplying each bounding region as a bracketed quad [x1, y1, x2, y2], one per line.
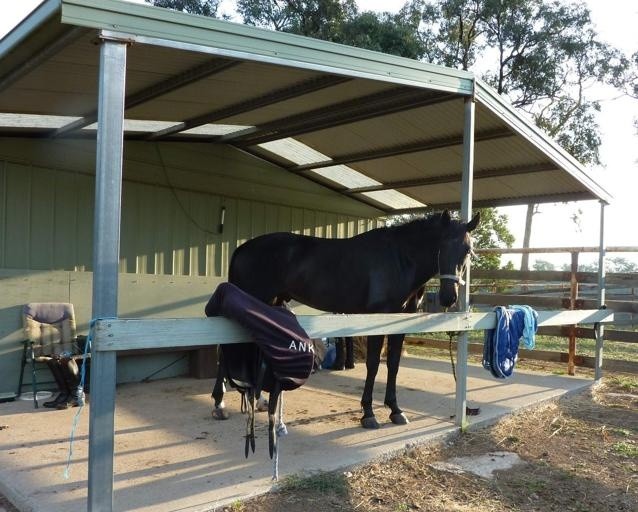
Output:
[42, 356, 86, 410]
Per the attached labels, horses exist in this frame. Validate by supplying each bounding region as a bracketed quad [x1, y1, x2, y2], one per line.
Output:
[212, 208, 480, 437]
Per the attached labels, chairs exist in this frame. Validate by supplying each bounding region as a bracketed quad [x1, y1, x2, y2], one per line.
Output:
[13, 300, 90, 407]
